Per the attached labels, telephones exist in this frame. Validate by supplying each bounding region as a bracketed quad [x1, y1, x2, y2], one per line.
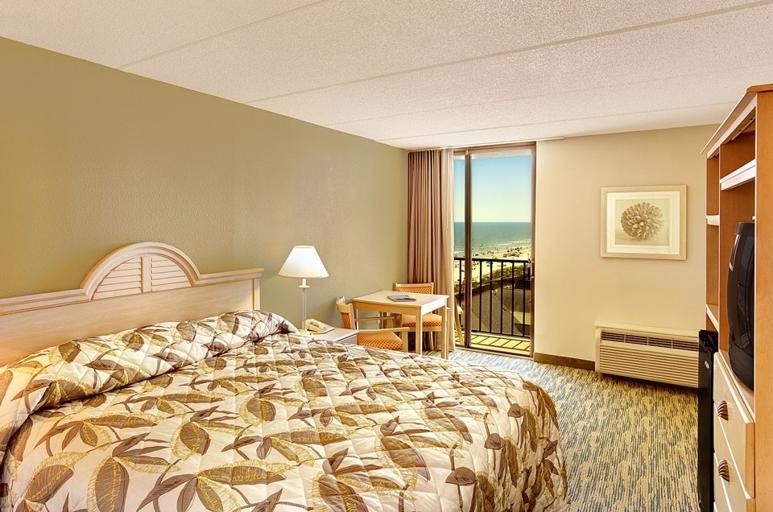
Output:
[302, 319, 335, 333]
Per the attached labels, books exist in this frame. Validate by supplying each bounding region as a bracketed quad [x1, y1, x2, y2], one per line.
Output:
[387, 294, 416, 302]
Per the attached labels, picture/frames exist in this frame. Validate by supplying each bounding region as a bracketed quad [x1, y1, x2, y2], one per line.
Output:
[600, 184, 688, 261]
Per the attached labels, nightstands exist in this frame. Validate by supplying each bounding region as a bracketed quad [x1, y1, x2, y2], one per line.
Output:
[309, 328, 359, 346]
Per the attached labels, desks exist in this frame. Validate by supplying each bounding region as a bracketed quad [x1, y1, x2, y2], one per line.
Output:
[353, 288, 449, 358]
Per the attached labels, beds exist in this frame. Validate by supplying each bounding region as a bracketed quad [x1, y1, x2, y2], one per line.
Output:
[0, 240, 543, 512]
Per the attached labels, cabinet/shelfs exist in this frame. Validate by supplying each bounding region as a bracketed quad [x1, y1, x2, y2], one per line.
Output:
[705, 83, 772, 512]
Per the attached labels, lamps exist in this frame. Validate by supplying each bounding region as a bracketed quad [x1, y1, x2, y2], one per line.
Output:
[278, 244, 330, 331]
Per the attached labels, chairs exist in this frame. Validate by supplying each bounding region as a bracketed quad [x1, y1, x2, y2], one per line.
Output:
[336, 282, 443, 353]
